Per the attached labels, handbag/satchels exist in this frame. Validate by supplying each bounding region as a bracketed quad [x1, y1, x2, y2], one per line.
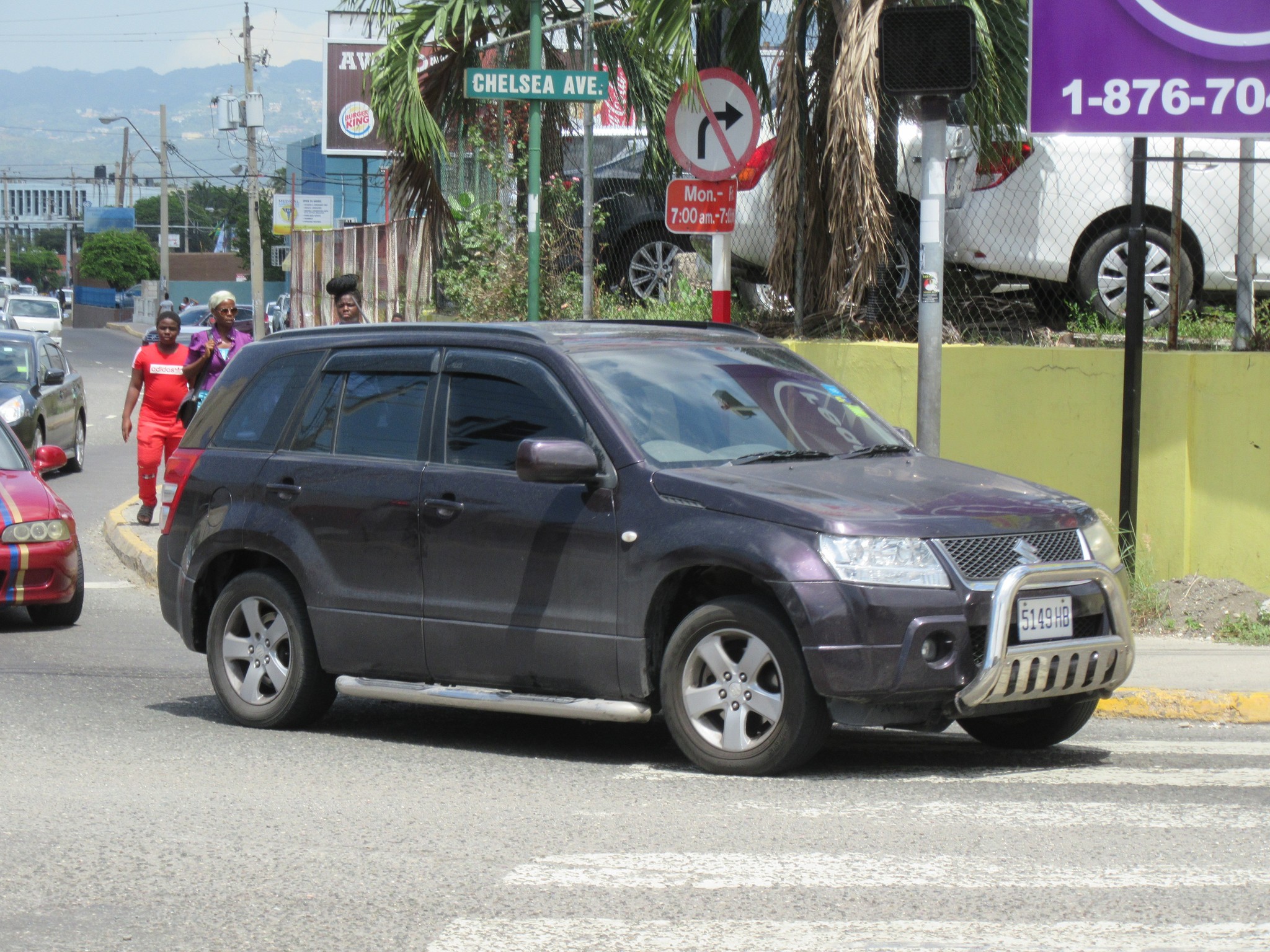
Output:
[176, 387, 198, 429]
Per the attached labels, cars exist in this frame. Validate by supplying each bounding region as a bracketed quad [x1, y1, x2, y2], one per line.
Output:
[0, 329, 87, 478]
[896, 115, 1269, 332]
[536, 139, 697, 310]
[0, 415, 85, 628]
[0, 313, 19, 330]
[19, 284, 39, 296]
[732, 89, 925, 304]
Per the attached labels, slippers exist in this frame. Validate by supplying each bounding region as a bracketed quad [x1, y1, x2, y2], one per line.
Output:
[137, 505, 153, 526]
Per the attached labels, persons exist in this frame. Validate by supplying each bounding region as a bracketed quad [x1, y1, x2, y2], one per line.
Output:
[157, 293, 173, 321]
[49, 287, 60, 300]
[12, 285, 16, 294]
[326, 274, 360, 325]
[392, 313, 403, 322]
[180, 297, 199, 310]
[182, 290, 253, 411]
[58, 288, 65, 313]
[122, 311, 188, 526]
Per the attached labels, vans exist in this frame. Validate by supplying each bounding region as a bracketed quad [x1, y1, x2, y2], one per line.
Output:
[3, 294, 63, 348]
[0, 277, 20, 308]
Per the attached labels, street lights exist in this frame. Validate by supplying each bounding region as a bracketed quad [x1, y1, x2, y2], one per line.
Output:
[228, 163, 264, 339]
[97, 114, 168, 312]
[114, 147, 160, 209]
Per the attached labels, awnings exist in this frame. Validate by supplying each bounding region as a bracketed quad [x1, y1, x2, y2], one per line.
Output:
[282, 251, 291, 271]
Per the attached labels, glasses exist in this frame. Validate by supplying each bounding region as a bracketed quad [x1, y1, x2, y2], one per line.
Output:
[214, 307, 239, 318]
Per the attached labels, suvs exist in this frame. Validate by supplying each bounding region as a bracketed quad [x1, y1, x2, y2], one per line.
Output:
[115, 284, 144, 310]
[265, 292, 290, 335]
[56, 289, 73, 307]
[141, 304, 271, 350]
[155, 326, 1136, 778]
